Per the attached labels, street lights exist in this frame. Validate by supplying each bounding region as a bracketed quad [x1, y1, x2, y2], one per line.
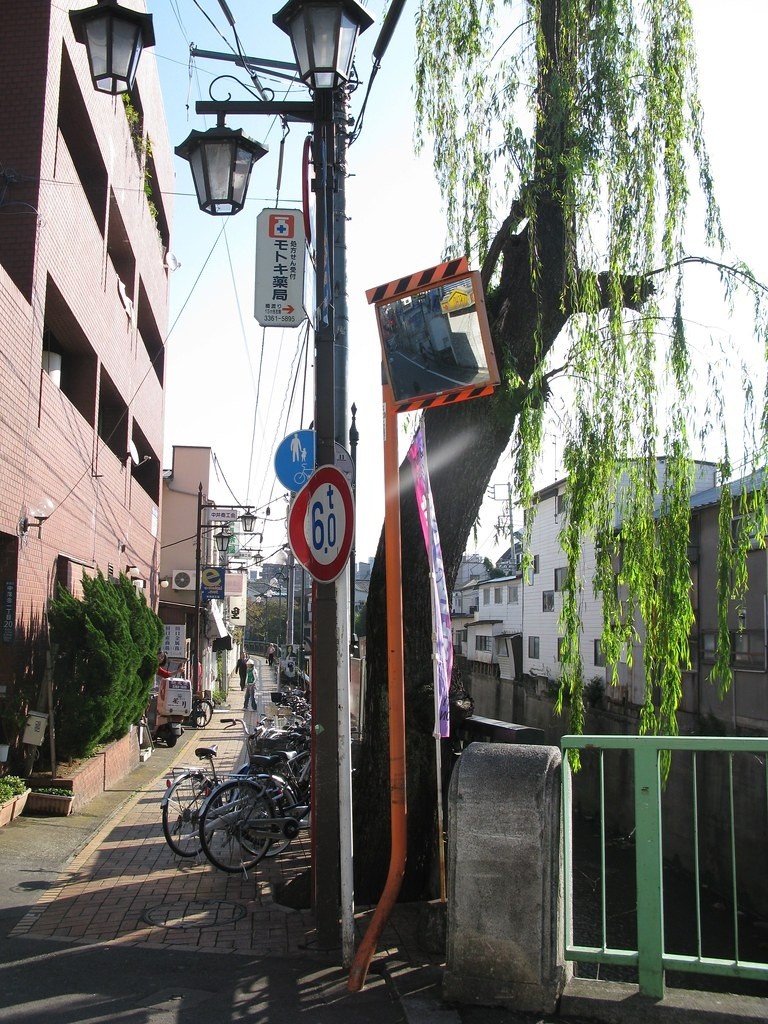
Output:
[256, 570, 289, 661]
[67, 0, 358, 964]
[191, 479, 257, 730]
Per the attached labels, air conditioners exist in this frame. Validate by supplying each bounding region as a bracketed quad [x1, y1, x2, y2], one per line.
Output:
[172, 570, 202, 591]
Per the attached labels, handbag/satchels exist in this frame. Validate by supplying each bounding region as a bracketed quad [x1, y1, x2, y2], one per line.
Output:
[266, 660, 267, 663]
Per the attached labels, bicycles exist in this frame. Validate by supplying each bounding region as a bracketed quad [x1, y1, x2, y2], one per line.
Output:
[192, 691, 214, 728]
[159, 687, 313, 880]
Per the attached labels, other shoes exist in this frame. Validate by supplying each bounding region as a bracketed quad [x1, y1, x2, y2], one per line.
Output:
[242, 708, 249, 711]
[249, 709, 256, 711]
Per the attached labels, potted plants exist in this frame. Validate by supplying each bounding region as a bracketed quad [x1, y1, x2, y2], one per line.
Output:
[0, 775, 32, 827]
[27, 788, 75, 816]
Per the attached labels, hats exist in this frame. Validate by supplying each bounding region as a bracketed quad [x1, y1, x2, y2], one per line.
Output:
[246, 659, 254, 664]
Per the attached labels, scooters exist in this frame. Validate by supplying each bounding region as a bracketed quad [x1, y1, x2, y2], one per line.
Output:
[150, 693, 184, 748]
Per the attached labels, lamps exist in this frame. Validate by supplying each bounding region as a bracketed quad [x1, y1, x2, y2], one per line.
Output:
[159, 579, 169, 588]
[24, 498, 54, 539]
[126, 565, 139, 576]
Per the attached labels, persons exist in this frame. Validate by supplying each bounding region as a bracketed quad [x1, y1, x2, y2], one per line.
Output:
[285, 645, 296, 671]
[154, 648, 186, 678]
[235, 643, 274, 711]
